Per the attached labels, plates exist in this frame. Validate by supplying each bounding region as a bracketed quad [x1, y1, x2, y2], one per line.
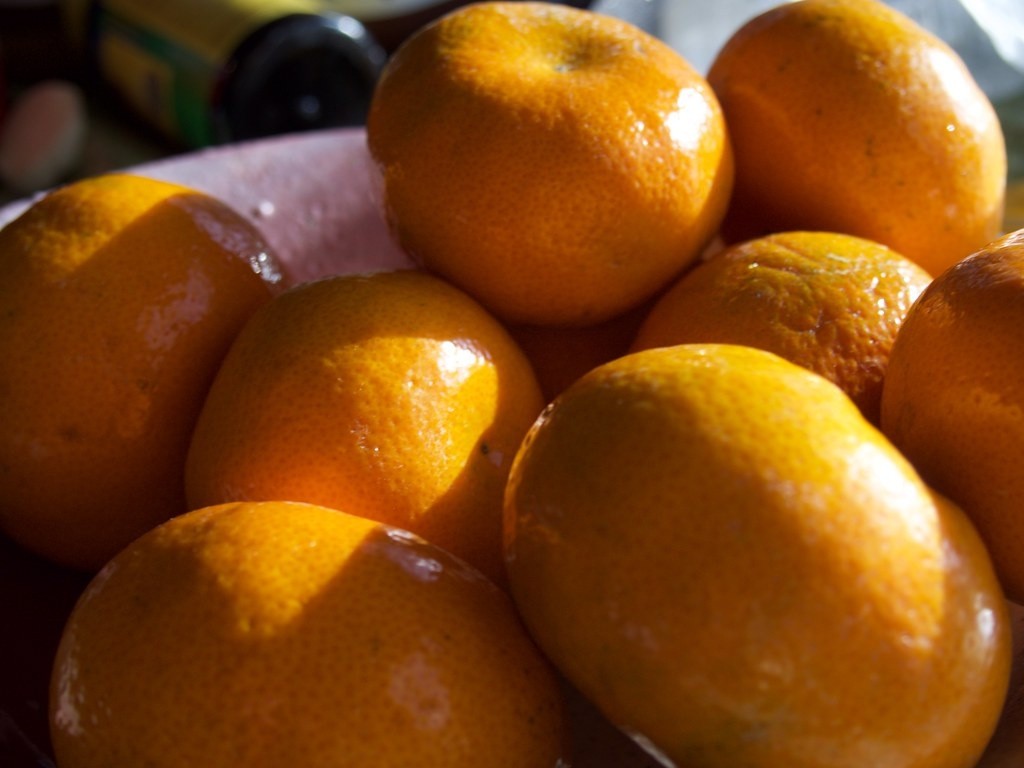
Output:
[0, 120, 1023, 768]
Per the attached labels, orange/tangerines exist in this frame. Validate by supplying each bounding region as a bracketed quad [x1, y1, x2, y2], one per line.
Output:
[0, 1, 1024, 768]
